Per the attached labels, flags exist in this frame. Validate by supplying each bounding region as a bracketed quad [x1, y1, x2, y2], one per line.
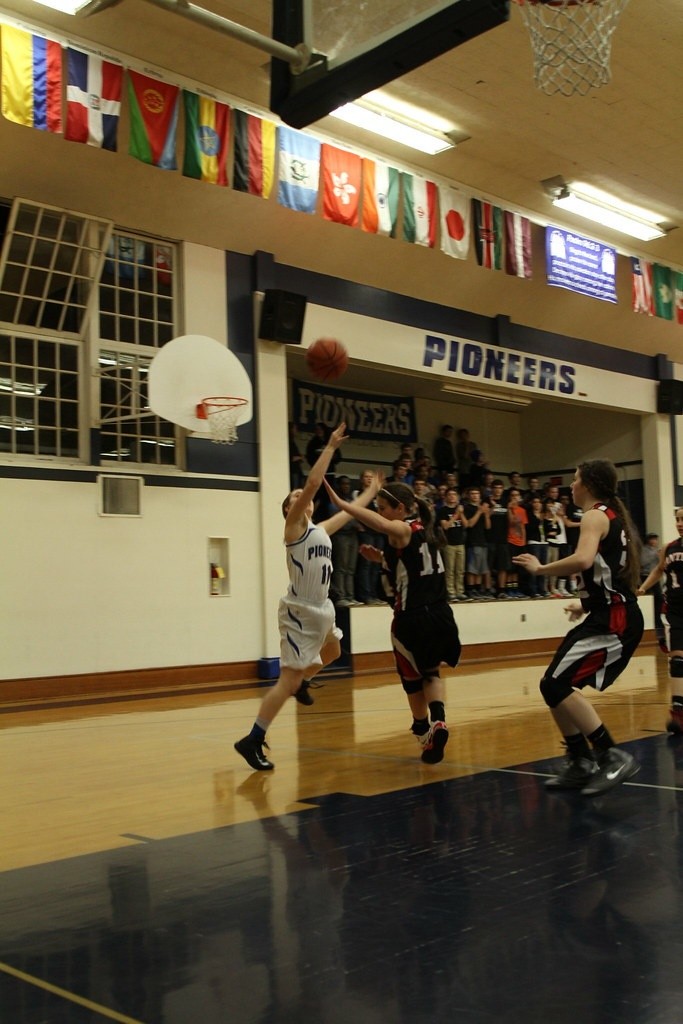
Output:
[630, 256, 656, 316]
[127, 69, 179, 170]
[402, 171, 437, 248]
[505, 210, 533, 279]
[361, 157, 400, 239]
[182, 89, 230, 187]
[64, 47, 124, 153]
[232, 108, 276, 200]
[653, 264, 683, 324]
[276, 126, 321, 215]
[438, 186, 471, 260]
[0, 22, 63, 134]
[322, 143, 361, 228]
[472, 198, 503, 270]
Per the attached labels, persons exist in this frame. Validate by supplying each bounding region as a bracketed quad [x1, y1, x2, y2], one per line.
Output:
[640, 532, 664, 628]
[289, 422, 583, 604]
[636, 507, 683, 733]
[512, 461, 644, 799]
[234, 423, 386, 772]
[322, 475, 462, 765]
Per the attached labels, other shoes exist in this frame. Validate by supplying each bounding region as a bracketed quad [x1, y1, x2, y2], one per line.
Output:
[666, 709, 683, 738]
[375, 598, 389, 606]
[448, 588, 582, 601]
[367, 600, 382, 605]
[351, 599, 364, 606]
[335, 598, 355, 606]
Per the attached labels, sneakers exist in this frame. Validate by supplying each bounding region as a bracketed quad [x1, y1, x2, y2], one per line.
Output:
[235, 771, 275, 801]
[291, 679, 326, 706]
[417, 729, 432, 748]
[542, 745, 601, 788]
[231, 732, 274, 770]
[420, 719, 449, 764]
[581, 746, 641, 798]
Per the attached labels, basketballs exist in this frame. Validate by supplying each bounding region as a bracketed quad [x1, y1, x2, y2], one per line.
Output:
[308, 338, 349, 382]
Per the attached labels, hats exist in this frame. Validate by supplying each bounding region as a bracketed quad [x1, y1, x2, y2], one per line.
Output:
[646, 532, 659, 538]
